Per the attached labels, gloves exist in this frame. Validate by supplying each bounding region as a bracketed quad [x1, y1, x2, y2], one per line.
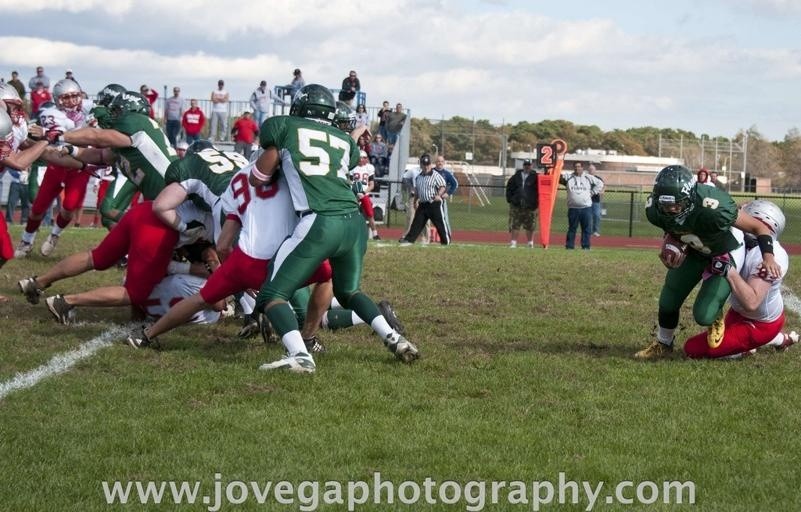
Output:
[82, 164, 114, 180]
[184, 220, 206, 239]
[705, 253, 731, 276]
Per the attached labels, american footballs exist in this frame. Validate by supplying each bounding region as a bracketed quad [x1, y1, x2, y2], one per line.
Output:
[661, 233, 689, 260]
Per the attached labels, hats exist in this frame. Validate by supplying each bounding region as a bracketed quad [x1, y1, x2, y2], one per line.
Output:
[420, 155, 431, 164]
[523, 159, 531, 165]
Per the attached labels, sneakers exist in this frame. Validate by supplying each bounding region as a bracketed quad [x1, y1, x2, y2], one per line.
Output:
[511, 240, 535, 249]
[236, 300, 421, 374]
[634, 311, 799, 362]
[12, 224, 153, 351]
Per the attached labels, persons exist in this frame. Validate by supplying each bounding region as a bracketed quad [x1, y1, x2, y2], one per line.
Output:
[1, 67, 797, 377]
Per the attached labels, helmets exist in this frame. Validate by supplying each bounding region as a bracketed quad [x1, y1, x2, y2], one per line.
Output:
[652, 165, 697, 224]
[741, 200, 786, 241]
[182, 139, 218, 155]
[250, 84, 355, 160]
[0, 79, 151, 163]
